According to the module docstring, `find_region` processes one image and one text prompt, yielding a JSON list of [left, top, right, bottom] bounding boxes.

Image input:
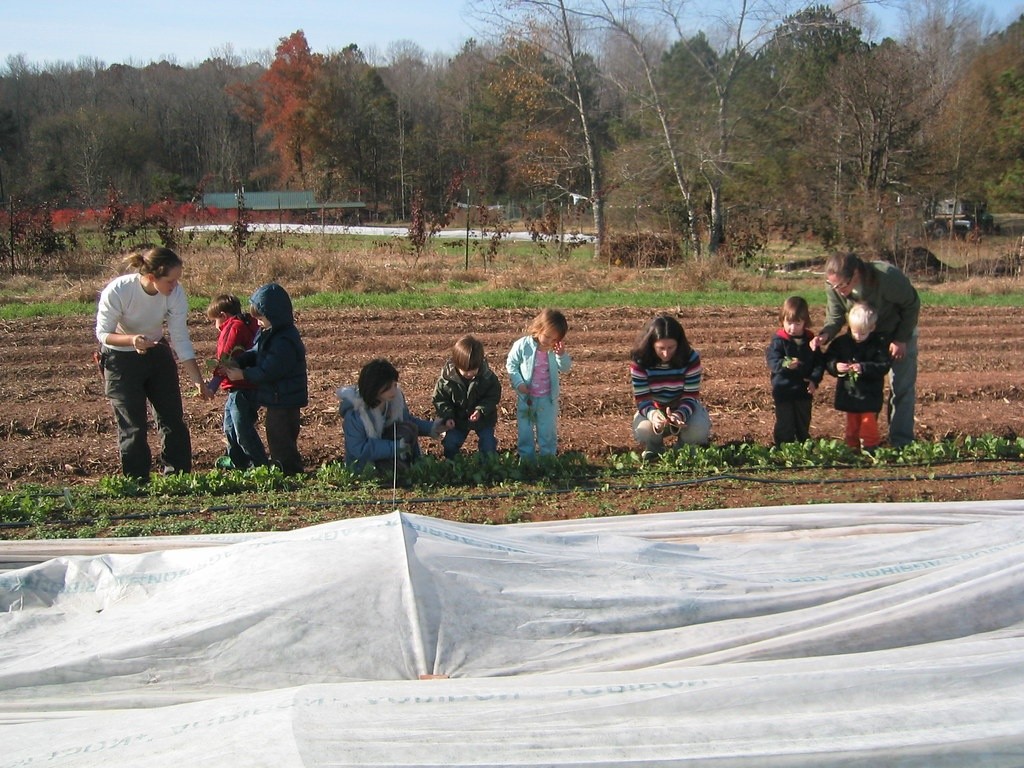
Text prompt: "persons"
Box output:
[[824, 303, 893, 448], [432, 337, 501, 460], [766, 296, 825, 452], [629, 316, 709, 458], [227, 284, 307, 478], [96, 248, 207, 480], [809, 252, 920, 449], [337, 358, 448, 472], [196, 295, 269, 469], [508, 310, 570, 460]]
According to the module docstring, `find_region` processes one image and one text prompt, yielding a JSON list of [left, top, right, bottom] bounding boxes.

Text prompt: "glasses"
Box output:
[[825, 274, 854, 289]]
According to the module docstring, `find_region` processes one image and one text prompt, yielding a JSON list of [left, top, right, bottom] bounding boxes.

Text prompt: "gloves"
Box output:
[[431, 418, 448, 433], [399, 437, 411, 455]]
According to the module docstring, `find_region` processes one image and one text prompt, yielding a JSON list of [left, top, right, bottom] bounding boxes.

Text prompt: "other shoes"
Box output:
[[214, 456, 238, 470], [862, 445, 881, 457], [848, 446, 860, 460]]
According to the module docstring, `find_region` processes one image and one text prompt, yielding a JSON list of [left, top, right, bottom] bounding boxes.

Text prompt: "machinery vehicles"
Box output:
[[917, 196, 1001, 238]]
